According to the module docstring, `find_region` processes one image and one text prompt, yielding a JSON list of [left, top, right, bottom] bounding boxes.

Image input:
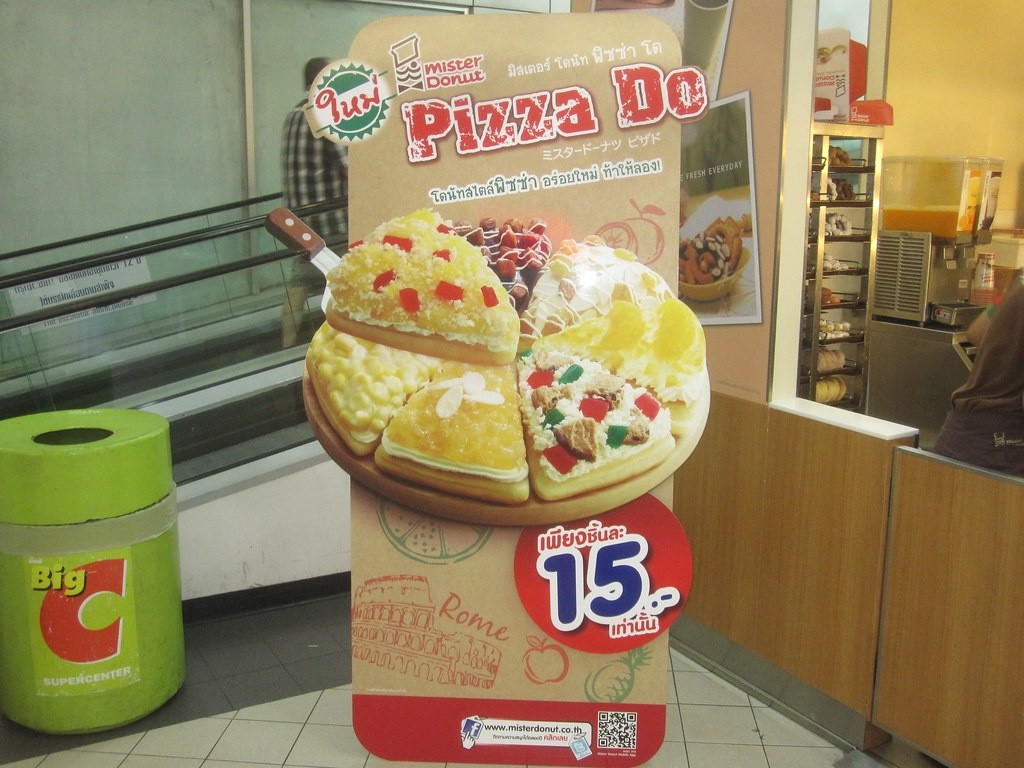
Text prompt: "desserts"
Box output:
[[679, 212, 752, 302]]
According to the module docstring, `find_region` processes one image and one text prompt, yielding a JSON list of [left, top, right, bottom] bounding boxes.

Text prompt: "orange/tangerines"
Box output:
[[959, 206, 976, 231]]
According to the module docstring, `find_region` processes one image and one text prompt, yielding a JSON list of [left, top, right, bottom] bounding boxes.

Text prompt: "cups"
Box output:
[[968, 252, 995, 309], [681, 0, 729, 72]]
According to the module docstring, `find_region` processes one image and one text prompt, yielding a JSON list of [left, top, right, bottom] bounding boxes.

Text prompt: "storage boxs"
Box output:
[[973, 234, 1024, 270]]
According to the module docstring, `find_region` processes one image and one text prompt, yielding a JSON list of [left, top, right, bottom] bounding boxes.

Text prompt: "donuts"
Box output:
[[816, 45, 848, 65], [799, 146, 854, 404]]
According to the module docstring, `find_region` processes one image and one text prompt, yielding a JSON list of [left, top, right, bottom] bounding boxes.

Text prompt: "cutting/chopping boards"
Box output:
[[301, 328, 712, 526]]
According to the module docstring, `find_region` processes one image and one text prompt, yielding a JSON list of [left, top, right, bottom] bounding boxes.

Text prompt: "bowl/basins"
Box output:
[[677, 247, 751, 301]]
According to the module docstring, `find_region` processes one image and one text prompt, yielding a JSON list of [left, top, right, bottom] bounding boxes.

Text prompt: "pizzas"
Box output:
[[306, 209, 708, 506]]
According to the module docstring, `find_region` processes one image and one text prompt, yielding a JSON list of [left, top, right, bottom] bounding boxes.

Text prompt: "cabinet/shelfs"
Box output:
[[796, 117, 886, 419]]
